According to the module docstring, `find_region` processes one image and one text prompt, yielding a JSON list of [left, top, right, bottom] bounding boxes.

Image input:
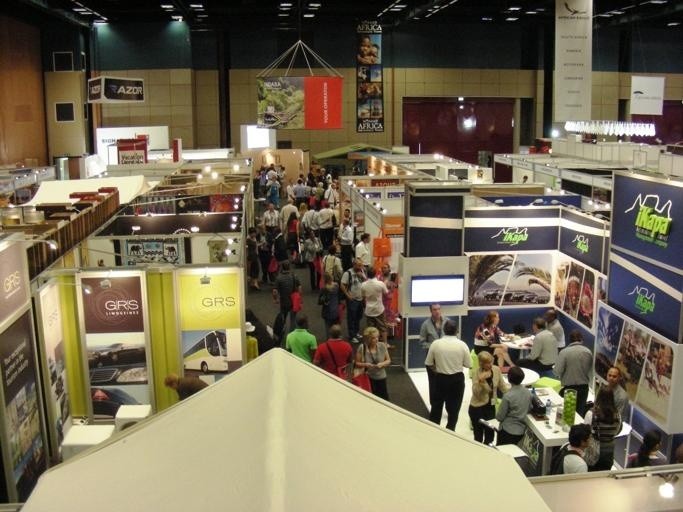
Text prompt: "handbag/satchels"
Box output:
[[337, 301, 348, 325], [267, 254, 280, 274], [336, 363, 351, 379], [350, 371, 372, 393], [331, 266, 343, 282], [290, 289, 303, 313]]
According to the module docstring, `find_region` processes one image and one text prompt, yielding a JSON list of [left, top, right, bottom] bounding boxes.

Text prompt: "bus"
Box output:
[[183, 329, 230, 377]]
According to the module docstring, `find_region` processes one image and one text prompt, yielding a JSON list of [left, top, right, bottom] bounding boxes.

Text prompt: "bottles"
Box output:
[[530, 387, 535, 394], [544, 399, 552, 416]]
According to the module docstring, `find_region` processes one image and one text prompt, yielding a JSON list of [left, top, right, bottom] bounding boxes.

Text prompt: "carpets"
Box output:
[[407, 368, 620, 470]]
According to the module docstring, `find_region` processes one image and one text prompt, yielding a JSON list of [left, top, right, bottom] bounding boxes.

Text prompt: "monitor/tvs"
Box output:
[[411, 274, 464, 306]]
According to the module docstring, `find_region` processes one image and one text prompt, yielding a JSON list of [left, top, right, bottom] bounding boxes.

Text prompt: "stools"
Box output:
[[615, 422, 632, 439], [491, 442, 529, 467], [479, 416, 500, 447]]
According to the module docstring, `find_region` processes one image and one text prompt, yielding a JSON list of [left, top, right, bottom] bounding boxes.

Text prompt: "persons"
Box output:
[[543, 308, 568, 349], [513, 317, 560, 374], [604, 365, 631, 422], [162, 374, 209, 401], [552, 328, 594, 418], [263, 99, 281, 124], [625, 429, 668, 469], [549, 422, 595, 478], [418, 300, 461, 414], [582, 388, 624, 473], [472, 310, 516, 368], [357, 33, 382, 123], [467, 350, 508, 445], [495, 367, 533, 448], [243, 157, 399, 405], [423, 319, 473, 433]]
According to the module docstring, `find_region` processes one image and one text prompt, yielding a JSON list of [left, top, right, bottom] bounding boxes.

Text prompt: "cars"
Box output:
[[483, 288, 539, 303], [88, 362, 149, 416], [49, 359, 68, 419]]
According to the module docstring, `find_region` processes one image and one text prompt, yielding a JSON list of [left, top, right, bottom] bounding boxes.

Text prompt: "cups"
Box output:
[[555, 407, 563, 425]]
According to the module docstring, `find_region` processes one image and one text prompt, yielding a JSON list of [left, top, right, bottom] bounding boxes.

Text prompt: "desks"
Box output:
[[518, 386, 584, 475], [504, 331, 537, 358]]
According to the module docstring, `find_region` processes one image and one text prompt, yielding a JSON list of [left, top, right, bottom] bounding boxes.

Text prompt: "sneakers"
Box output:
[[348, 332, 363, 345]]
